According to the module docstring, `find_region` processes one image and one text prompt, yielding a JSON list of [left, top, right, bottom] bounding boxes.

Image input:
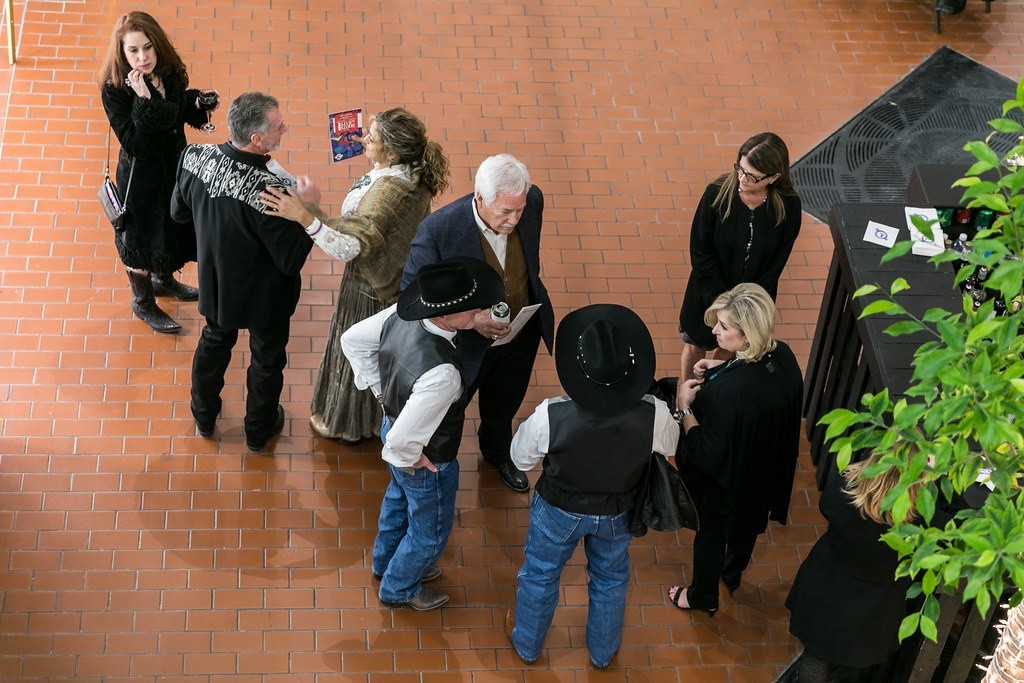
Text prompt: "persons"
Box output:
[[340, 255, 504, 612], [503, 304, 680, 670], [170, 93, 321, 453], [784, 422, 1000, 683], [402, 154, 555, 491], [668, 283, 804, 617], [100, 11, 220, 333], [257, 107, 449, 441], [678, 132, 801, 383]]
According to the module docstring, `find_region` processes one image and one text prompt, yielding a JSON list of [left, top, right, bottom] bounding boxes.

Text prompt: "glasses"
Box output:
[[734, 163, 771, 183]]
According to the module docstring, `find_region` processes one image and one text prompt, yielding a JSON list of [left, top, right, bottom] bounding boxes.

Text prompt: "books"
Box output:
[[328, 109, 365, 162]]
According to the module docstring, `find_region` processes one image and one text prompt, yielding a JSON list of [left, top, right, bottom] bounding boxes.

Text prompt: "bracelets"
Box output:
[[680, 408, 693, 419]]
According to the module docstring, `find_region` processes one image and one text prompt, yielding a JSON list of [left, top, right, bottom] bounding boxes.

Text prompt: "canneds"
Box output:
[[492, 301, 510, 318]]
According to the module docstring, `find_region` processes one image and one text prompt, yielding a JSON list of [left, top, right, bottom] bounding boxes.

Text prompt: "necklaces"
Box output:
[[155, 76, 160, 89], [738, 188, 767, 202]]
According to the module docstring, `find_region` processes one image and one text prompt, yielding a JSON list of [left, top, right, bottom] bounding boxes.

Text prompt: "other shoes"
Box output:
[[504, 608, 517, 640], [199, 431, 212, 437], [246, 405, 284, 451], [311, 414, 361, 446]]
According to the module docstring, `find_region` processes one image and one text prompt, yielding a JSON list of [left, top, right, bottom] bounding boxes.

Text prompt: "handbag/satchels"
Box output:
[[98, 178, 126, 229]]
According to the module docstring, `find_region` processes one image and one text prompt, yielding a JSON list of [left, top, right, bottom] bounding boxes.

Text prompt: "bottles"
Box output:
[[943, 232, 1020, 317]]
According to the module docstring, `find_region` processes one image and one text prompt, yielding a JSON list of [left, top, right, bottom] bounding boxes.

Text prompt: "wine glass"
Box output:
[[198, 88, 218, 134]]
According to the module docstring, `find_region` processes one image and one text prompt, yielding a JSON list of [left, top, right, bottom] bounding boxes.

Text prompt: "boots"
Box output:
[[150, 270, 199, 301], [126, 268, 182, 332]]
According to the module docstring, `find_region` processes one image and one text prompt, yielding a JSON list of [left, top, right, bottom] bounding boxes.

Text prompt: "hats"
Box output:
[[554, 304, 656, 416], [395, 256, 505, 322]]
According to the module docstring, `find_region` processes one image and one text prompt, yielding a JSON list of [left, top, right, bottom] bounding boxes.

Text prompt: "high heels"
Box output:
[[724, 582, 740, 596], [668, 585, 718, 617]]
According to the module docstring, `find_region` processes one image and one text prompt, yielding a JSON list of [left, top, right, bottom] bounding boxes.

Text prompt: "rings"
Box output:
[[492, 334, 497, 340]]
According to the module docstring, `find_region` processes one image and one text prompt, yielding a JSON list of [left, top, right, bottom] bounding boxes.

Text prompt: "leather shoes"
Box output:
[[372, 566, 441, 582], [500, 462, 531, 491], [406, 586, 449, 611]]
